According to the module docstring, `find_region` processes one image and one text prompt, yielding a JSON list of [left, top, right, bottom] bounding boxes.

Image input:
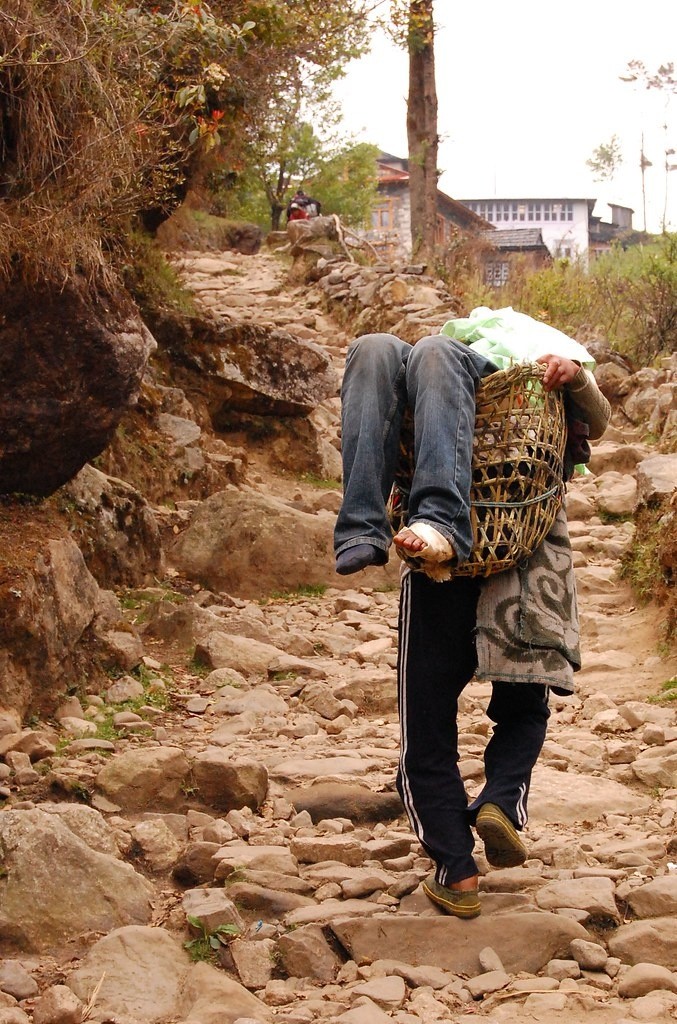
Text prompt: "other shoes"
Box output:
[[423, 871, 482, 919], [476, 802, 528, 868]]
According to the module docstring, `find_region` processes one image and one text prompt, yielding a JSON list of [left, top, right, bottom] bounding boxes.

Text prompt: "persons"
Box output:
[[287, 191, 322, 221], [334, 306, 612, 918]]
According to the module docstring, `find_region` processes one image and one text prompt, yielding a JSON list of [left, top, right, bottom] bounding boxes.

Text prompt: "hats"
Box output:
[[291, 202, 300, 209]]
